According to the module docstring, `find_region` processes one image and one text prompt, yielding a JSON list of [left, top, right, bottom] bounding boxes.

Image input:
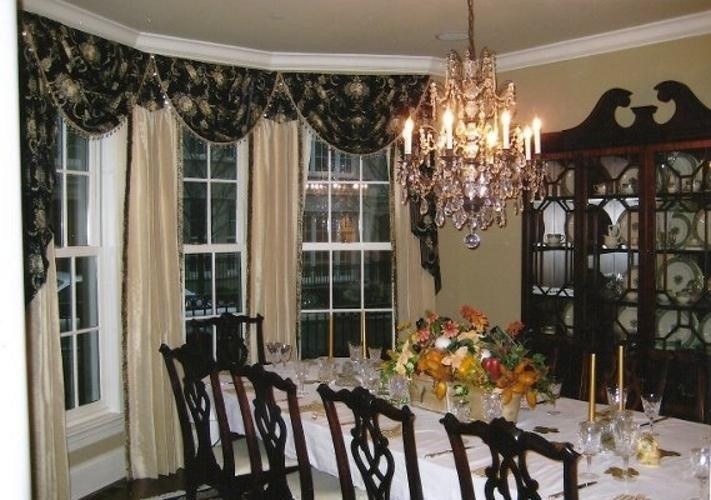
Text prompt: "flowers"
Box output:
[[374, 304, 562, 407]]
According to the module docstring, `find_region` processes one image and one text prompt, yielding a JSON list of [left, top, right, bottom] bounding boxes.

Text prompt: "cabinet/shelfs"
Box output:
[[521, 80, 711, 424]]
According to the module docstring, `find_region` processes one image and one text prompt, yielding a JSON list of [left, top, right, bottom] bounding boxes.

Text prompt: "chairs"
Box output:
[[159, 312, 710, 499]]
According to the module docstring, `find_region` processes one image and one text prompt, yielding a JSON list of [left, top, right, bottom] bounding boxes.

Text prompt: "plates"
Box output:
[[561, 152, 711, 350], [544, 242, 566, 247]]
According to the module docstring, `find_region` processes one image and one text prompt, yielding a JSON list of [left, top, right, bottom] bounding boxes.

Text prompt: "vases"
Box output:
[[409, 373, 523, 424]]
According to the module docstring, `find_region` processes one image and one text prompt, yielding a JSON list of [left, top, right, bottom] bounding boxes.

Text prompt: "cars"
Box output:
[[53, 269, 239, 351]]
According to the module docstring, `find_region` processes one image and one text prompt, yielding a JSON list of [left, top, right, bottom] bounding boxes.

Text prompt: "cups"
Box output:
[[451, 398, 471, 423], [481, 393, 503, 420], [367, 346, 382, 363], [548, 233, 567, 244]]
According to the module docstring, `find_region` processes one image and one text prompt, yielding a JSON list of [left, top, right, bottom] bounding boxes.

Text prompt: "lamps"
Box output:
[[395, 1, 547, 248]]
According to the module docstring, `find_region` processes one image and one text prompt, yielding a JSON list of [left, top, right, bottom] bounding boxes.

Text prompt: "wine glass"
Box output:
[[579, 423, 602, 480], [360, 365, 381, 397], [348, 343, 361, 375], [544, 381, 561, 415], [606, 385, 630, 422], [278, 344, 292, 374], [690, 448, 711, 500], [318, 357, 331, 384], [265, 342, 278, 371], [388, 377, 408, 408], [612, 421, 638, 481], [294, 360, 310, 397], [641, 393, 663, 436]]
